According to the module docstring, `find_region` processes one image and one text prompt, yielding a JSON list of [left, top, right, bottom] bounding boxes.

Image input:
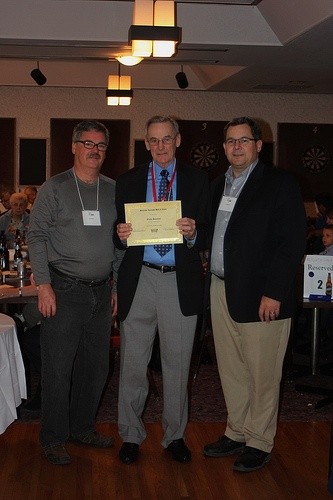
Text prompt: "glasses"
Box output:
[[225, 137, 255, 147], [146, 131, 179, 146], [73, 140, 106, 151]]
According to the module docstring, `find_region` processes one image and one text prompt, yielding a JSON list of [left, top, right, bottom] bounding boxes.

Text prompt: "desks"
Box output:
[[0, 267, 41, 303], [0, 309, 30, 441]]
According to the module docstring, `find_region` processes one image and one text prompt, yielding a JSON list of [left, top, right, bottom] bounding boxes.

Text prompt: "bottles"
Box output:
[[0, 230, 9, 270], [14, 229, 23, 271]]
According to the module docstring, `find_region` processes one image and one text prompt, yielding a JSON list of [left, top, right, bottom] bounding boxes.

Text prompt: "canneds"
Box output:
[[17, 261, 25, 276]]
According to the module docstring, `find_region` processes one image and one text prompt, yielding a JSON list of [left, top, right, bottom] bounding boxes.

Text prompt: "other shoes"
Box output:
[[169, 438, 192, 462], [202, 435, 246, 457], [233, 446, 272, 472], [39, 439, 72, 465], [70, 427, 115, 448], [119, 442, 139, 464]]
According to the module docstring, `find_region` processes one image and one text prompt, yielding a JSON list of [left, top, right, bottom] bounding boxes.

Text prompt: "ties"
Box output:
[[153, 169, 173, 257]]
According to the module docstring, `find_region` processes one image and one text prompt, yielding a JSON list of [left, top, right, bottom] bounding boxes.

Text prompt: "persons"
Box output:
[[306, 192, 333, 254], [209, 117, 306, 472], [27, 120, 117, 465], [112, 115, 214, 463], [0, 184, 37, 249]]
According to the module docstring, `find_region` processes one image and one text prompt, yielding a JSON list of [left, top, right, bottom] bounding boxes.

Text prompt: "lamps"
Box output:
[[114, 55, 144, 66], [128, 0, 182, 57], [105, 57, 132, 107], [30, 59, 47, 85], [175, 64, 188, 89]]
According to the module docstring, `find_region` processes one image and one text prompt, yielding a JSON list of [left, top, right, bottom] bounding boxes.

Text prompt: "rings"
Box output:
[[270, 312, 275, 314]]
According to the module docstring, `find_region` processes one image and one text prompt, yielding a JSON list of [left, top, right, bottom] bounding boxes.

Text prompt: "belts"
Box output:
[[211, 273, 226, 280], [142, 261, 175, 274], [47, 264, 110, 285]]
